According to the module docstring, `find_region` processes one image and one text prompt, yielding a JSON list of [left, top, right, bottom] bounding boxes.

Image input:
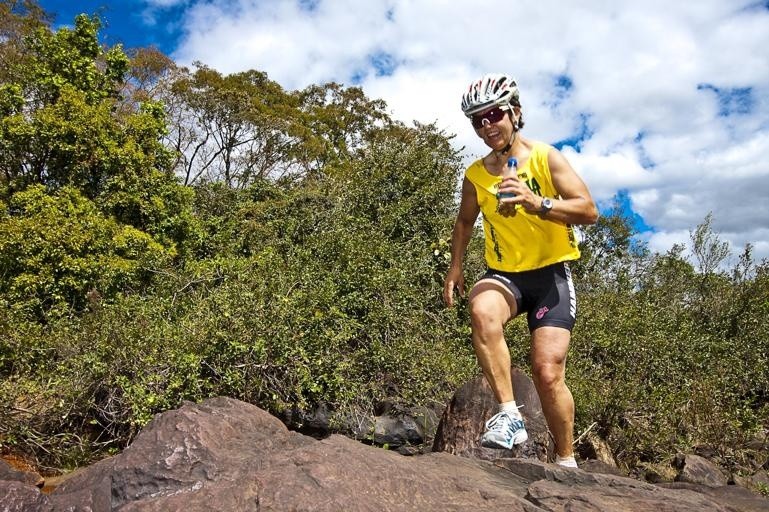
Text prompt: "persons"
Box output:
[[444, 74, 600, 469]]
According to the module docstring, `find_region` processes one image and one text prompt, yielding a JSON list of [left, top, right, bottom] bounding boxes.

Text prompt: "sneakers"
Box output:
[[480, 412, 529, 451]]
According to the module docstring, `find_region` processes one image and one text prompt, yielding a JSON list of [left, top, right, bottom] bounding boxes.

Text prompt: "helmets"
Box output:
[[460, 72, 520, 116]]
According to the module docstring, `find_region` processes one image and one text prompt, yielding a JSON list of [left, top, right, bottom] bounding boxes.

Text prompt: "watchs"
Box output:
[[539, 195, 554, 219]]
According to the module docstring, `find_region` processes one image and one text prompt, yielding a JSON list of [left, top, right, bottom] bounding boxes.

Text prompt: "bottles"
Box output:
[[496, 157, 518, 217]]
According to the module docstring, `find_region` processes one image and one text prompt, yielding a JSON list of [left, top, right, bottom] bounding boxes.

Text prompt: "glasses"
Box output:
[[471, 106, 515, 129]]
